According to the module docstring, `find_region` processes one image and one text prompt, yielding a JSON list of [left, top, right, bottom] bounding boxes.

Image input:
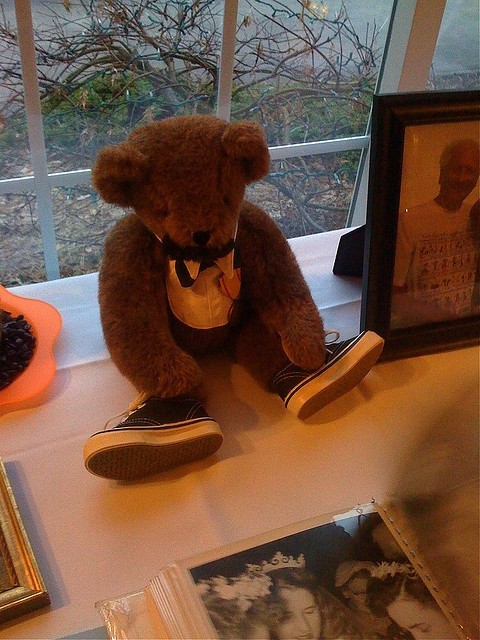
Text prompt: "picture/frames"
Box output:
[[0, 462, 52, 621], [332, 88, 480, 359]]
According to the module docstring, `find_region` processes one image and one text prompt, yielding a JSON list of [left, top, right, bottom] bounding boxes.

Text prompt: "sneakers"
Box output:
[[268, 329, 385, 421], [82, 390, 223, 481]]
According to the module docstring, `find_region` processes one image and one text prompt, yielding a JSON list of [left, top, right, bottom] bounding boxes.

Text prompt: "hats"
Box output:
[[334, 562, 377, 588]]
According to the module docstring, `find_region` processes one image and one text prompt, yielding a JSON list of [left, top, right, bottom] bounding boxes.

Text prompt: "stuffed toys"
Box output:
[[81, 113, 387, 483]]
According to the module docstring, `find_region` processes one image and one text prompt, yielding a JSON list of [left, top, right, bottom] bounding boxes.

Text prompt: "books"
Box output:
[[93, 499, 475, 640]]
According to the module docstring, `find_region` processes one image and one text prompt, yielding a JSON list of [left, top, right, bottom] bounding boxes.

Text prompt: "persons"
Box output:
[[244, 549, 377, 640], [388, 139, 480, 328], [367, 561, 460, 639], [332, 558, 412, 640], [195, 572, 277, 640]]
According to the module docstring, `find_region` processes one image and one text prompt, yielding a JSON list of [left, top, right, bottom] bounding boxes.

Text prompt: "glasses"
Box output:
[[347, 587, 367, 597]]
